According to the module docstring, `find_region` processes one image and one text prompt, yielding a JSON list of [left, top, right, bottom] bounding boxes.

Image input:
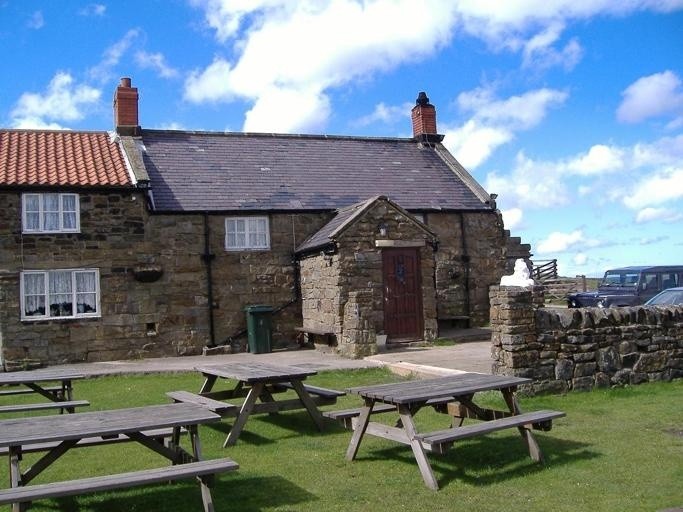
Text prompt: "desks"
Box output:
[[0, 368, 84, 413], [193, 359, 330, 449], [0, 402, 221, 488], [346, 373, 541, 489]]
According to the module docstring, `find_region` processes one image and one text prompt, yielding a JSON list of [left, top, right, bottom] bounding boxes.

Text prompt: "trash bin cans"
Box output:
[[244, 305, 273, 353]]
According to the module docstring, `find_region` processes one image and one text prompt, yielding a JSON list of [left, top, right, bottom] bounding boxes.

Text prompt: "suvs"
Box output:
[[566, 264, 683, 309]]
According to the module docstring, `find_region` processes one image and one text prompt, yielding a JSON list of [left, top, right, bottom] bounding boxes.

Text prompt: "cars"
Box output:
[[644, 286, 683, 306]]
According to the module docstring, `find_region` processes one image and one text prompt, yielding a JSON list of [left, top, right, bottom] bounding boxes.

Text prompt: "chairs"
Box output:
[[647, 278, 657, 288], [664, 279, 675, 289], [631, 276, 639, 282]]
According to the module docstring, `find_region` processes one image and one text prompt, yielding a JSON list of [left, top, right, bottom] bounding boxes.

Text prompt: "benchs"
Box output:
[[1, 387, 67, 395], [322, 396, 457, 430], [413, 408, 568, 456], [166, 390, 237, 420], [0, 400, 90, 414], [273, 383, 346, 403], [1, 426, 188, 460], [1, 456, 239, 512]]
